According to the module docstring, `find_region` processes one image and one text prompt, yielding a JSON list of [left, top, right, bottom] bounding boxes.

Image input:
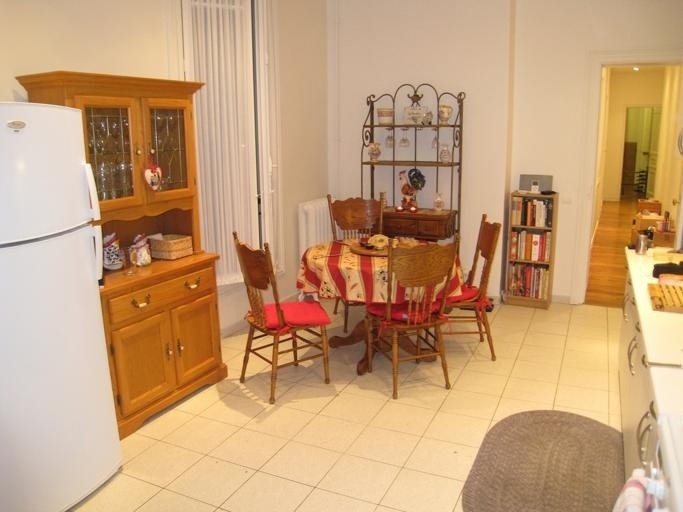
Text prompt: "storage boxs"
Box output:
[[636, 199, 661, 215], [636, 212, 664, 231], [652, 226, 675, 248], [631, 228, 649, 248]]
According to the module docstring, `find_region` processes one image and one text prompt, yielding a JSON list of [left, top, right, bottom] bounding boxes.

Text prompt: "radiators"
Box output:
[[299, 198, 366, 301]]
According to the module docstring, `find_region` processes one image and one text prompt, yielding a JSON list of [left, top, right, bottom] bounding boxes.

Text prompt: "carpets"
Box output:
[[462, 410, 624, 512]]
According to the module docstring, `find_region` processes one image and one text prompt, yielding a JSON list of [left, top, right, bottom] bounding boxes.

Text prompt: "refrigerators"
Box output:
[[0, 101, 126, 512]]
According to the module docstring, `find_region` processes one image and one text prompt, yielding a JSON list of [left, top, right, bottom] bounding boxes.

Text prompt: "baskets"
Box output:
[[150, 234, 193, 261]]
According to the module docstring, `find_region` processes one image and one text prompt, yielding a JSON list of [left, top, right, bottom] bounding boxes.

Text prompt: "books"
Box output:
[[507, 197, 553, 300]]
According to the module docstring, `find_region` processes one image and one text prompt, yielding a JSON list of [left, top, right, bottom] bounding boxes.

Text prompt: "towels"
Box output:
[[611, 475, 656, 512], [611, 469, 667, 512]]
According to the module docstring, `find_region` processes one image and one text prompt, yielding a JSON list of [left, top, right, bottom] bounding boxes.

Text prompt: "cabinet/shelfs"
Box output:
[[619, 246, 683, 512], [358, 83, 464, 243], [100, 253, 226, 441], [502, 192, 559, 310], [15, 71, 205, 270]]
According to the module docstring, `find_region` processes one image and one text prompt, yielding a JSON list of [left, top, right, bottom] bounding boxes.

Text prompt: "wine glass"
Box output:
[[386, 128, 392, 147], [399, 128, 408, 147], [432, 128, 439, 148]]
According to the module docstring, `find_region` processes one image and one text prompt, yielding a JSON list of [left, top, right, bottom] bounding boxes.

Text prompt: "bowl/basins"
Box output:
[[360, 242, 367, 246], [366, 244, 373, 249]]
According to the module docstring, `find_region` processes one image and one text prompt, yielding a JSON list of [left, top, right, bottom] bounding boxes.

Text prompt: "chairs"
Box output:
[[233, 233, 330, 403], [327, 193, 398, 333], [367, 236, 457, 399], [439, 213, 502, 361]]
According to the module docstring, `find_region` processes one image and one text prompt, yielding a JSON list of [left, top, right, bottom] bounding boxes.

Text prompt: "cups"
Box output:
[[392, 239, 399, 248], [636, 236, 647, 254], [121, 246, 136, 276]]
